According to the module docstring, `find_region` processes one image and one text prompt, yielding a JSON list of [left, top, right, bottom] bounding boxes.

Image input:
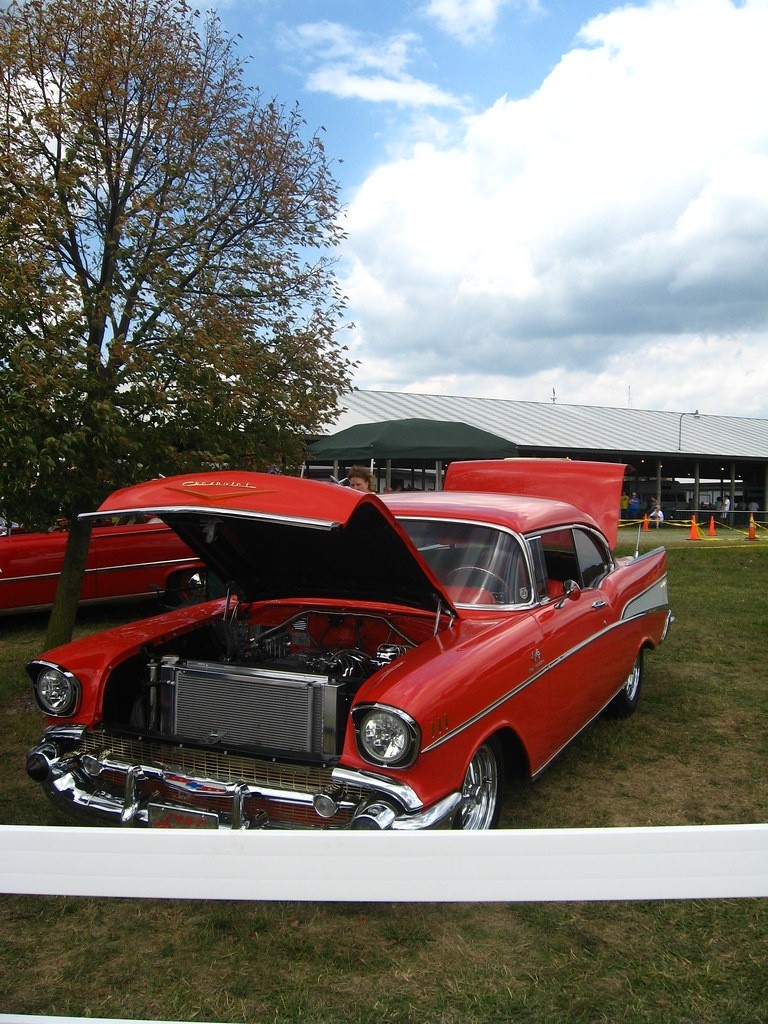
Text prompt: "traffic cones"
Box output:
[[706, 513, 719, 537], [685, 514, 703, 541], [745, 513, 761, 542], [640, 512, 652, 532]]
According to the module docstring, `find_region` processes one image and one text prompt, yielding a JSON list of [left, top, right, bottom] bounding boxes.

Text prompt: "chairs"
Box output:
[[480, 550, 527, 603]]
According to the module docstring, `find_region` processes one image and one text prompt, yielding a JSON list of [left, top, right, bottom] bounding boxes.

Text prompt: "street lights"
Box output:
[[678, 409, 700, 452]]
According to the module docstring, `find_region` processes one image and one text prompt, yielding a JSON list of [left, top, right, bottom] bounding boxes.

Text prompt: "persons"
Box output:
[[382, 485, 760, 526], [349, 466, 373, 494]]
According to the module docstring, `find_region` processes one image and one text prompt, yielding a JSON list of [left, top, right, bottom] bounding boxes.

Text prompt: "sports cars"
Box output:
[[0, 457, 210, 613], [26, 455, 675, 831]]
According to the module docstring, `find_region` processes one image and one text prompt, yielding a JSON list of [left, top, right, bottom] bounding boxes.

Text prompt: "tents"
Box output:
[[305, 419, 519, 493]]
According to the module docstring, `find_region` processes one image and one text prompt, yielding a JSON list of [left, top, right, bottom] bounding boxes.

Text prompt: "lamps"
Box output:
[[679, 410, 700, 450]]
